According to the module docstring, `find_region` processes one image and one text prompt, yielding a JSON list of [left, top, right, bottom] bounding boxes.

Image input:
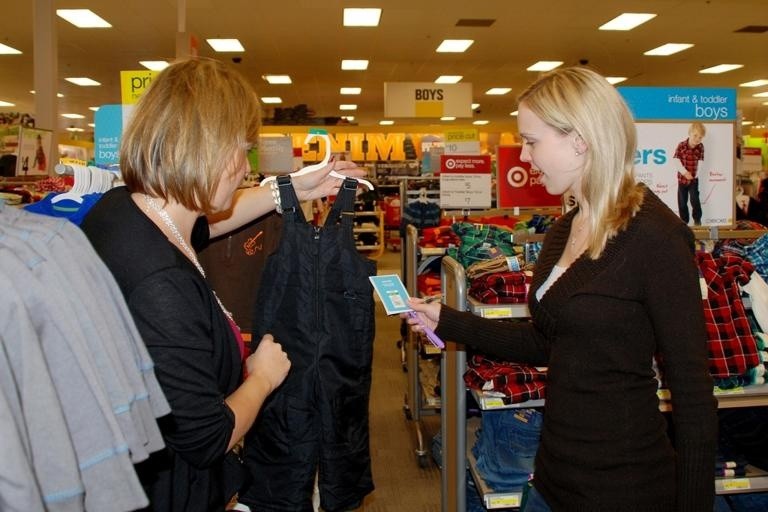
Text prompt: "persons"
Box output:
[[397, 61, 721, 512], [672, 122, 707, 225], [31, 133, 46, 171], [76, 54, 367, 512]]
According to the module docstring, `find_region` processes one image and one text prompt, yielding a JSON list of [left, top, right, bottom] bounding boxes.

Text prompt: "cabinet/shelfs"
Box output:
[[399, 214, 768, 509], [307, 164, 442, 262], [734, 171, 767, 203]]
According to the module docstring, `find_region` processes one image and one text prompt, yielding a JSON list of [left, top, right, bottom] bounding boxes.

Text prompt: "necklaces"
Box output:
[[137, 192, 233, 319], [568, 217, 590, 252]]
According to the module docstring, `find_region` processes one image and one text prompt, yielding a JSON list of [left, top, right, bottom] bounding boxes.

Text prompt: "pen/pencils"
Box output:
[[408, 312, 445, 350]]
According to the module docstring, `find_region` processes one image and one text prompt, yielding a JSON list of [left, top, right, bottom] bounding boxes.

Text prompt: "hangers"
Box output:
[[259, 128, 379, 192], [45, 163, 115, 209]]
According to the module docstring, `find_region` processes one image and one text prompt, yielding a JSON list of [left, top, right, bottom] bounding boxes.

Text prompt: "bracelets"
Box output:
[[268, 178, 283, 215]]
